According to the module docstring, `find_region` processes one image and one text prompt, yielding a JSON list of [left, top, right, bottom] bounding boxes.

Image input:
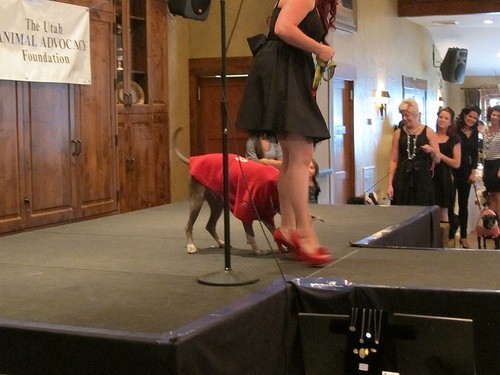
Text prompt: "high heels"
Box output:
[[459, 238, 471, 249], [274, 227, 306, 261], [291, 232, 332, 266]]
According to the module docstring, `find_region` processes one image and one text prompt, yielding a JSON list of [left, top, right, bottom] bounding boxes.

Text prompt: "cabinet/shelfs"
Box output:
[[0, 0, 170, 234]]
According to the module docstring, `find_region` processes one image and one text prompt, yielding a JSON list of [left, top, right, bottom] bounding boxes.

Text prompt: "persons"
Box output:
[[455, 105, 479, 249], [307, 158, 320, 203], [481, 105, 500, 218], [435, 107, 461, 222], [385, 98, 440, 206], [235, 0, 337, 266], [242, 133, 283, 169]]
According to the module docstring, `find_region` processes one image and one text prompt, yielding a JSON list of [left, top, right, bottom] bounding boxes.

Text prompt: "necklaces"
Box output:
[[404, 127, 421, 160]]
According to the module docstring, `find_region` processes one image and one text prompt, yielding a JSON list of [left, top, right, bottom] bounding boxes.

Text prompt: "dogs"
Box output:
[[172, 125, 325, 256], [476, 209, 500, 250], [474, 189, 491, 209], [347, 190, 392, 206]]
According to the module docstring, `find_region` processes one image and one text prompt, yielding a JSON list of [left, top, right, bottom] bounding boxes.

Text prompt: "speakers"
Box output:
[[440, 48, 468, 84], [168, 0, 211, 21]]
